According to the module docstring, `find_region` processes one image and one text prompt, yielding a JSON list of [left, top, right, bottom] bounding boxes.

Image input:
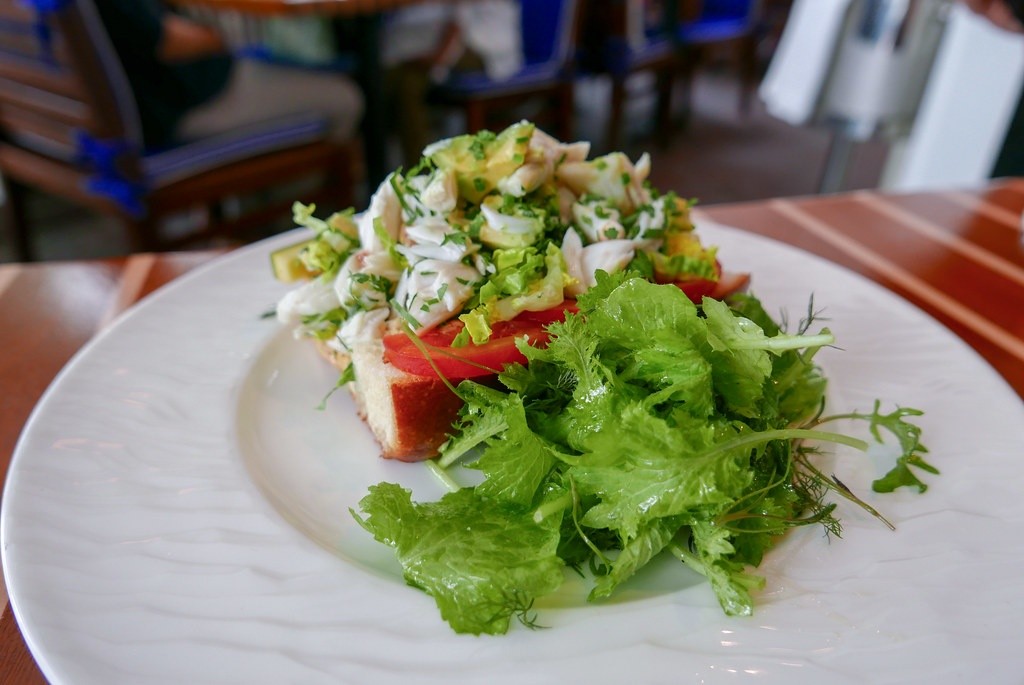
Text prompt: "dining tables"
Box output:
[[0, 179, 1024, 684]]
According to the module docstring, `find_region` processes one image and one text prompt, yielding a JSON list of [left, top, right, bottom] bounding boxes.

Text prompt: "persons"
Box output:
[[386, 0, 579, 175], [90, 0, 361, 235]]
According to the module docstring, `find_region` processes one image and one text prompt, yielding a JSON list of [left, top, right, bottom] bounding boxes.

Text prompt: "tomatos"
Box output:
[[379, 320, 551, 378], [514, 302, 587, 325], [653, 273, 753, 304]]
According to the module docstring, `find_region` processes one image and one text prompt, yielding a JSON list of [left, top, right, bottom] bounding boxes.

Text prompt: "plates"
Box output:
[[0, 213, 1024, 685]]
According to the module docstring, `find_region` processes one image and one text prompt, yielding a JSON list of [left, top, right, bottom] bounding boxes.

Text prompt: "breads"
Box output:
[[342, 339, 515, 462]]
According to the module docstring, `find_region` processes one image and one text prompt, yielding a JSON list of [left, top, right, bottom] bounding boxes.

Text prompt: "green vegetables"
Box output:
[[288, 126, 716, 405], [351, 268, 941, 640]]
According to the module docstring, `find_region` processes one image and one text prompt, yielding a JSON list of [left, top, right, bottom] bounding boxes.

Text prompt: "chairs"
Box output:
[[0, 0, 363, 251], [368, 0, 764, 172]]
